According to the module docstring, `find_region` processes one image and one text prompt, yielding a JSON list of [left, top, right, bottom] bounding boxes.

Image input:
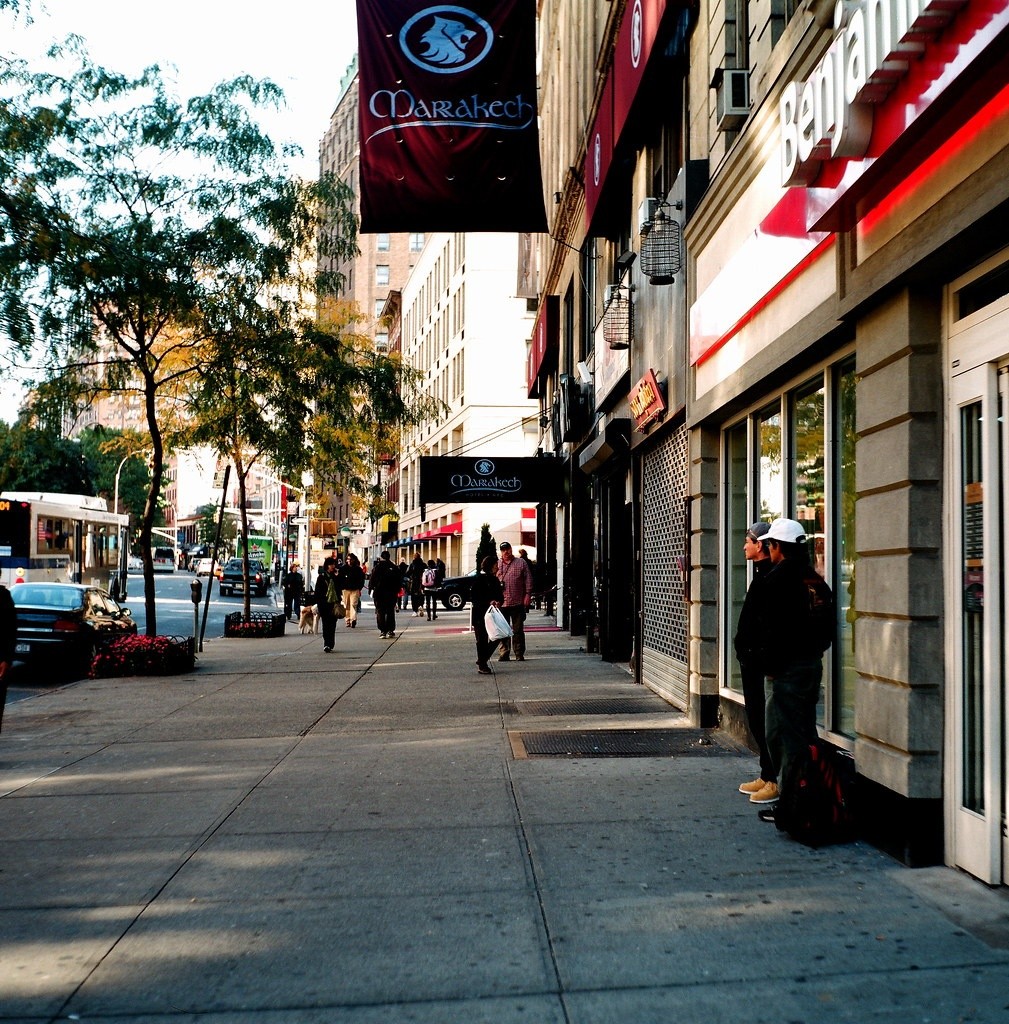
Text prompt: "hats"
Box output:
[[757, 517, 806, 543], [500, 542, 511, 550], [745, 521, 771, 541], [382, 551, 390, 560]]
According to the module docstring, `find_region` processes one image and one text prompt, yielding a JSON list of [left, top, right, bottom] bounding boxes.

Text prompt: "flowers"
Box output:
[[87, 633, 189, 680], [229, 620, 272, 639]]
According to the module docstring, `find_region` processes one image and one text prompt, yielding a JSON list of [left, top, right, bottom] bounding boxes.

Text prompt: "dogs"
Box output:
[[298, 604, 321, 636]]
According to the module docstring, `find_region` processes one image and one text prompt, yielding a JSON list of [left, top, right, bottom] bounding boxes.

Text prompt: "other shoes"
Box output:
[[758, 809, 775, 822], [427, 617, 431, 621], [476, 659, 479, 665], [418, 606, 424, 617], [432, 615, 438, 620], [324, 646, 330, 653], [379, 632, 386, 639], [412, 612, 418, 617], [351, 620, 356, 628], [386, 631, 395, 638], [346, 623, 350, 627], [498, 656, 510, 661], [478, 667, 491, 673], [516, 656, 524, 661]]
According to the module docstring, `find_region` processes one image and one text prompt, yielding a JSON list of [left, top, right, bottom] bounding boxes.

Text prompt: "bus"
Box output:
[[0, 492, 130, 603]]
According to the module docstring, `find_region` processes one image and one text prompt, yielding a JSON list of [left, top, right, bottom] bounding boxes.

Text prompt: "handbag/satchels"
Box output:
[[333, 603, 346, 619], [485, 605, 514, 642]]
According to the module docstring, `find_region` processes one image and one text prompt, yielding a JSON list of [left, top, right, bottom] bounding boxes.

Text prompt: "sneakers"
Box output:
[[739, 778, 765, 795], [749, 781, 780, 804]]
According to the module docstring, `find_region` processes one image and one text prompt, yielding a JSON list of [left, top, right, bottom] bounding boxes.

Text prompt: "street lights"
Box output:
[[289, 535, 296, 564], [341, 527, 351, 563]]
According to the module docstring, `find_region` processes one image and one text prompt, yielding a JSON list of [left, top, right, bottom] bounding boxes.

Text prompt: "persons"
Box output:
[[471, 557, 507, 674], [495, 543, 531, 661], [370, 549, 447, 639], [315, 555, 343, 653], [518, 549, 532, 573], [283, 563, 304, 620], [701, 518, 835, 804], [340, 552, 363, 628], [0, 567, 18, 730]]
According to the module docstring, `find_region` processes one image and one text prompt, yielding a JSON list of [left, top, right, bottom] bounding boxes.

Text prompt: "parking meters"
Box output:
[[190, 580, 203, 653]]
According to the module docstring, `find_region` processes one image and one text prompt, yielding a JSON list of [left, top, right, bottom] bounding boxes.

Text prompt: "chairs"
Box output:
[[26, 591, 45, 604]]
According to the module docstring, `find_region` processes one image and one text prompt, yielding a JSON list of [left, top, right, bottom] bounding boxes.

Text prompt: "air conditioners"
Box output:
[[637, 197, 658, 236], [716, 70, 751, 133]]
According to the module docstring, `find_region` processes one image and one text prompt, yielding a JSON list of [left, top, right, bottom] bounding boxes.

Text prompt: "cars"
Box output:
[[436, 568, 486, 611], [188, 558, 269, 597], [7, 582, 137, 681]]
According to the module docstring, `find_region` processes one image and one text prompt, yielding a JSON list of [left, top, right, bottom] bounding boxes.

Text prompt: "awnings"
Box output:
[[386, 521, 462, 548]]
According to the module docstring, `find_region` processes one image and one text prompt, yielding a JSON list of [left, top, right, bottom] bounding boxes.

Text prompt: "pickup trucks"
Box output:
[[152, 547, 174, 572]]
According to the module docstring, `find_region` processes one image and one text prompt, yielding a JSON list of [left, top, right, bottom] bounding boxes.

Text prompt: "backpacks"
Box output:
[[421, 568, 435, 587], [775, 744, 856, 848]]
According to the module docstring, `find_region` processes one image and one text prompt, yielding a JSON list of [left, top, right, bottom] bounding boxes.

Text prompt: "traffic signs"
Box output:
[[288, 515, 308, 525]]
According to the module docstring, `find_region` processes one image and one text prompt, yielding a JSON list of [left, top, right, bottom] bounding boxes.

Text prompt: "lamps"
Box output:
[[638, 200, 683, 286], [602, 284, 635, 351]]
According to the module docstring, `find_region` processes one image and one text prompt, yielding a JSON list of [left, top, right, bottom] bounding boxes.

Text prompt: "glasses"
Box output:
[[745, 529, 758, 540]]
